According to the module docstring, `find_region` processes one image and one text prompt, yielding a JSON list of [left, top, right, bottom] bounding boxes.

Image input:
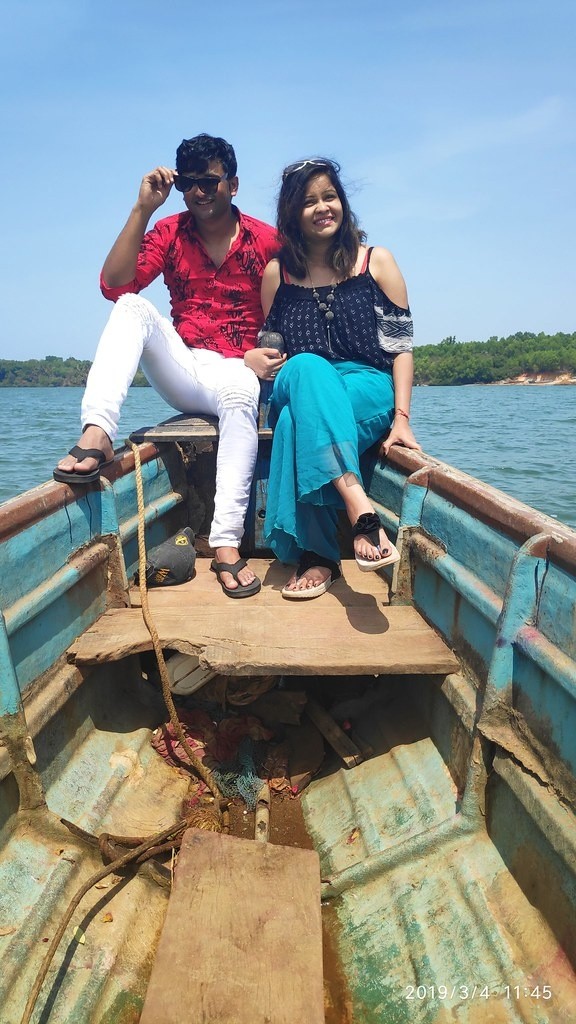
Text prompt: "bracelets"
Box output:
[[394, 407, 410, 420]]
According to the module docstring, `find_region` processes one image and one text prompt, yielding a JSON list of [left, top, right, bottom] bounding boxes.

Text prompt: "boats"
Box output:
[[0, 412, 574, 1022]]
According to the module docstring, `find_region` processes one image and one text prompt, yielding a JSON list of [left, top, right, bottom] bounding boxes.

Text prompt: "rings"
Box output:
[[270, 372, 276, 378]]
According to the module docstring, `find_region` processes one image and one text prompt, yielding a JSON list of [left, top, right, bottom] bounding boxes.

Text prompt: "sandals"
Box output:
[[282, 554, 342, 598], [52, 445, 114, 483], [351, 512, 401, 572], [211, 556, 261, 598]]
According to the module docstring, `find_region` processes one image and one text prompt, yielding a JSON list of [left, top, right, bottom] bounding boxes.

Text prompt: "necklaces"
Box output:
[[303, 259, 340, 352]]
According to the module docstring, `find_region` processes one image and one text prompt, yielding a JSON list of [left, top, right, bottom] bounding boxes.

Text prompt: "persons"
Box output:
[[243, 157, 424, 598], [53, 132, 285, 598]]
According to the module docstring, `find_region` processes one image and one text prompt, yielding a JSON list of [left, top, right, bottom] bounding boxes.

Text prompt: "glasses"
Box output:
[[282, 160, 333, 181], [173, 169, 229, 194]]
